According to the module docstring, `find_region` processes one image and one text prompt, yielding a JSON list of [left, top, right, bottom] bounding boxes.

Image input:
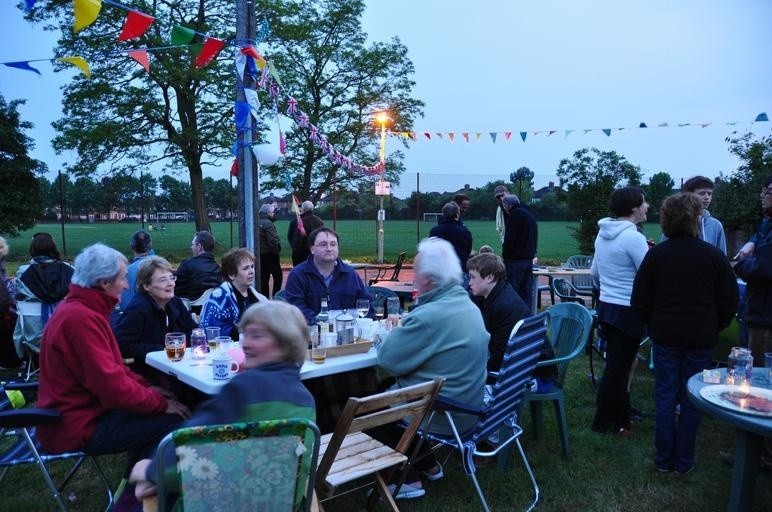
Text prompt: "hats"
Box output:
[[495, 186, 507, 197]]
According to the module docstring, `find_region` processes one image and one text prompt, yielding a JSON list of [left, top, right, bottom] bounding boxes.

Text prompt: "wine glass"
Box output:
[[373, 306, 384, 329], [356, 298, 370, 318]]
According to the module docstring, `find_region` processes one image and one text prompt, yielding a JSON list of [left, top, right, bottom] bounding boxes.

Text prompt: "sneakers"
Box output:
[[593, 408, 644, 436], [474, 454, 498, 466]]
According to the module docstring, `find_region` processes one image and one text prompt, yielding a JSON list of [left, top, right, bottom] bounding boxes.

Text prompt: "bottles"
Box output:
[[316, 296, 334, 333], [726, 346, 753, 397], [190, 328, 207, 360]]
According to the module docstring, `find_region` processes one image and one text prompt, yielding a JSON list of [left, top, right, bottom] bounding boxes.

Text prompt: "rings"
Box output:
[[175, 400, 179, 407]]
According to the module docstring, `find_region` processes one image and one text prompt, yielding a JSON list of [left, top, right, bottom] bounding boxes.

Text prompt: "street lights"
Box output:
[[373, 110, 389, 261]]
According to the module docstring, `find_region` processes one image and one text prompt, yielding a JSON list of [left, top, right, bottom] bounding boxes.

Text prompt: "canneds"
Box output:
[[728, 346, 753, 379], [387, 296, 400, 315]]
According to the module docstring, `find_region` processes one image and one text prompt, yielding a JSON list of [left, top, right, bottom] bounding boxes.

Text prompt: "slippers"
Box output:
[[424, 461, 443, 480], [367, 483, 425, 501]]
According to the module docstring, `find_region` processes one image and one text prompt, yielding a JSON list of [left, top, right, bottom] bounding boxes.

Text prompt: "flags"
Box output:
[[258, 70, 384, 176]]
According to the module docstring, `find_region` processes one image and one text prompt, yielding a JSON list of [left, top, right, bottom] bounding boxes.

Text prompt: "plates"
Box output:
[[699, 384, 771, 416]]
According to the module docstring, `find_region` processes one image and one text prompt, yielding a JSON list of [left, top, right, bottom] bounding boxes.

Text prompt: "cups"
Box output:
[[765, 352, 771, 380], [165, 332, 187, 364], [214, 336, 234, 353], [212, 356, 240, 379], [387, 296, 399, 326], [311, 331, 327, 363], [206, 326, 222, 352], [325, 334, 337, 346]]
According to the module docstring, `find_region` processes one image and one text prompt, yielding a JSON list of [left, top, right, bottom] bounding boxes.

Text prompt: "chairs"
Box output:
[[0, 252, 600, 512]]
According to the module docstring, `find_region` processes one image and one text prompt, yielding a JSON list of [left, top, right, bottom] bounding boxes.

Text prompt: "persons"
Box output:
[[258, 203, 282, 299], [36, 243, 194, 490], [427, 184, 540, 307], [466, 253, 558, 469], [590, 187, 651, 438], [286, 201, 324, 267], [658, 176, 726, 256], [114, 255, 210, 407], [632, 191, 740, 474], [728, 177, 771, 366], [283, 228, 375, 392], [0, 212, 274, 372], [129, 300, 310, 512], [367, 237, 491, 499]]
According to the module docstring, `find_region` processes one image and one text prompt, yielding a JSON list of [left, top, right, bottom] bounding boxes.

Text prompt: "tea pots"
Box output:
[[335, 309, 362, 345]]
